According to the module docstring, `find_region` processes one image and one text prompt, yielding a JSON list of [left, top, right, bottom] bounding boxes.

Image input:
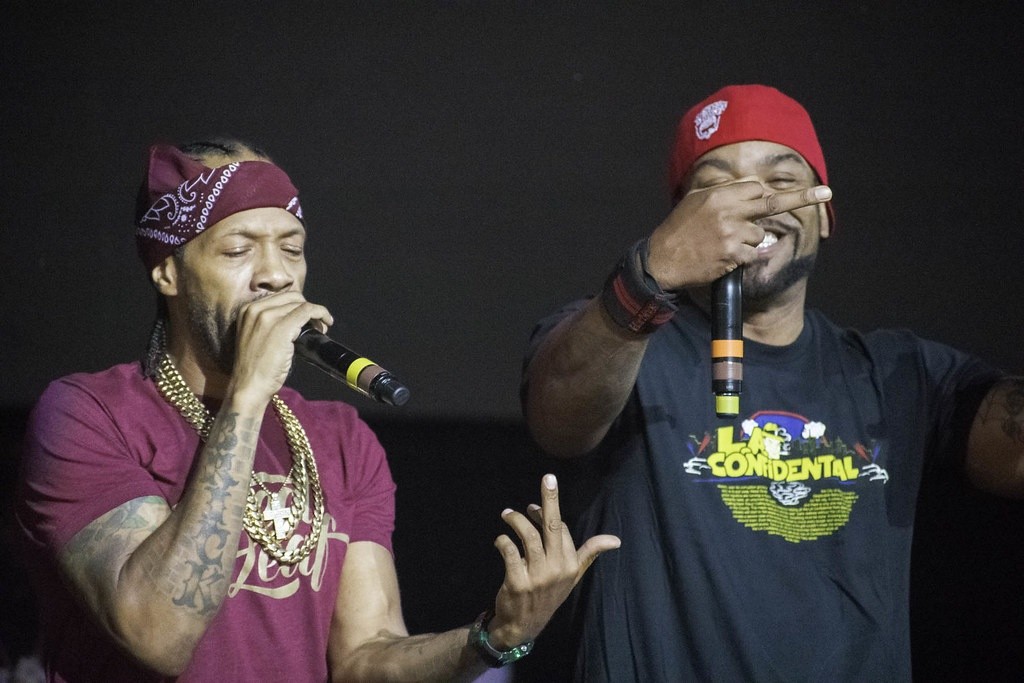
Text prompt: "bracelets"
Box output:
[[602, 237, 689, 335]]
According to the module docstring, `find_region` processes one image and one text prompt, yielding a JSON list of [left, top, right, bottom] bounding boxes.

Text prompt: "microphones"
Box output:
[[710, 263, 748, 418], [293, 324, 411, 406]]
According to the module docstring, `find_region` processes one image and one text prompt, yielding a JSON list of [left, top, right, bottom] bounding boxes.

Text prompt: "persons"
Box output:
[[14, 139, 622, 683], [520, 85, 1024, 683]]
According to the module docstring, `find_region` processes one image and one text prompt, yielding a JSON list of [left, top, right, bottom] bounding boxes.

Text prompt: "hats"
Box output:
[[667, 85, 838, 238]]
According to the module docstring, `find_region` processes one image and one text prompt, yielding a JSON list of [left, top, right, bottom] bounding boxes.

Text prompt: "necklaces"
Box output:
[[154, 352, 324, 566]]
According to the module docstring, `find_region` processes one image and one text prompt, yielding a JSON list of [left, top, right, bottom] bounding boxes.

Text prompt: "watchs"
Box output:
[[472, 610, 535, 668]]
